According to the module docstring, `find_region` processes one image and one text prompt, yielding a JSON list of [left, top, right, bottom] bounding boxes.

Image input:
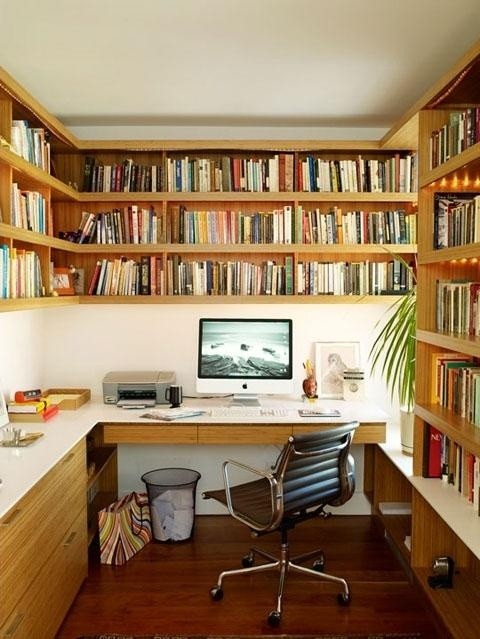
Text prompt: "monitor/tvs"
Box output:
[[194, 317, 294, 399]]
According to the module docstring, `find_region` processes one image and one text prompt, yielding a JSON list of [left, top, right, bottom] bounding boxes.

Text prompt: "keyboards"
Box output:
[[209, 406, 291, 419]]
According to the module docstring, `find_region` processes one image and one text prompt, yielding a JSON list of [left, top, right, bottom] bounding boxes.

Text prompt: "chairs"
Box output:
[[200, 422, 360, 628]]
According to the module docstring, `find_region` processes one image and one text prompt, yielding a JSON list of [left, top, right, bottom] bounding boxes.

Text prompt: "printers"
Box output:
[[103, 372, 176, 408]]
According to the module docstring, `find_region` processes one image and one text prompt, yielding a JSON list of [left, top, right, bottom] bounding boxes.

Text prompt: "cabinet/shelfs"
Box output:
[[0, 434, 90, 638]]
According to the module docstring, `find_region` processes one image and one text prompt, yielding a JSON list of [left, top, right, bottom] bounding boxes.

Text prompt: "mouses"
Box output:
[[315, 407, 333, 414]]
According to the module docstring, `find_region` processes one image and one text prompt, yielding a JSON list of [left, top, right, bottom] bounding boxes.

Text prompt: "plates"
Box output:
[[2, 433, 44, 446]]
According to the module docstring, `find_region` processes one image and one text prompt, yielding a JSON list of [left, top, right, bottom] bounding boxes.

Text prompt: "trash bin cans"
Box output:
[[141, 468, 201, 544]]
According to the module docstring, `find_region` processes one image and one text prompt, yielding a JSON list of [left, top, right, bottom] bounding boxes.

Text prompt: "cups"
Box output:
[[343, 368, 364, 402], [165, 385, 183, 408], [0, 428, 21, 443]]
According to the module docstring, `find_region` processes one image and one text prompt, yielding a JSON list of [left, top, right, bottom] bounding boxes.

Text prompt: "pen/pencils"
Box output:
[[303, 359, 314, 378]]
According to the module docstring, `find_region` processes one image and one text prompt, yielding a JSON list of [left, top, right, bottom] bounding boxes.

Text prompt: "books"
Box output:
[[8, 404, 59, 424], [140, 405, 206, 422], [296, 152, 416, 296], [7, 396, 52, 414], [168, 153, 295, 294], [0, 119, 49, 298], [75, 156, 164, 295], [417, 91, 478, 510], [298, 405, 342, 419]]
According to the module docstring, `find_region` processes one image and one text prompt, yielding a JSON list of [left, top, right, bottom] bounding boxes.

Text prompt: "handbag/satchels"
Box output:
[[96, 491, 154, 567]]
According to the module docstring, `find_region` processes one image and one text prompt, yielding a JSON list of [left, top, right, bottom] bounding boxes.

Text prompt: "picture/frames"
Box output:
[[314, 341, 361, 400]]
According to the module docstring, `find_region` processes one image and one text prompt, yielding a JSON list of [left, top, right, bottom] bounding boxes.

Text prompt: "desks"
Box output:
[[1, 395, 386, 547]]
